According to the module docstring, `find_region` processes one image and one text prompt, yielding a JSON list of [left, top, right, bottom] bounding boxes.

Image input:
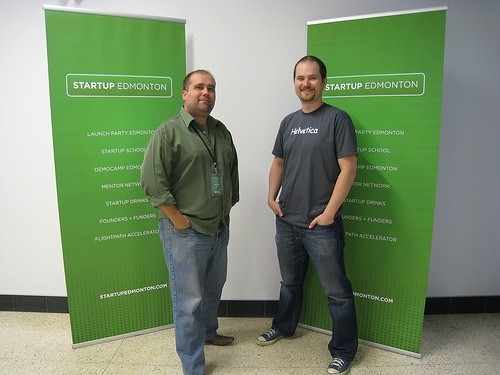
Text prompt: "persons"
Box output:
[[258, 56, 359, 374], [142, 70, 239, 374]]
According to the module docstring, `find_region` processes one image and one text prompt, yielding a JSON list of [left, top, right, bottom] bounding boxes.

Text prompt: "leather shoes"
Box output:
[[202, 334, 234, 346]]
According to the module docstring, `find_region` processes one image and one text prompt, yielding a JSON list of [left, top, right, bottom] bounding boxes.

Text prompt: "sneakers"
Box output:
[[327, 356, 352, 375], [255, 328, 284, 346]]
